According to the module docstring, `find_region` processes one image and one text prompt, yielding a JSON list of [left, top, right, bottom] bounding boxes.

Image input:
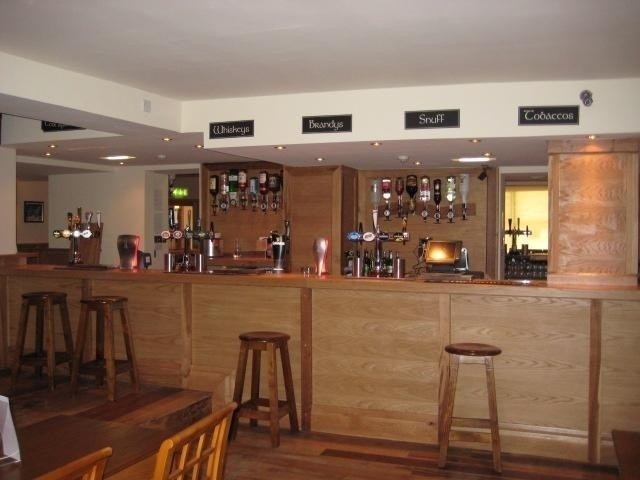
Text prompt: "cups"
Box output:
[[163, 252, 176, 271], [392, 258, 405, 278], [205, 238, 215, 259], [273, 241, 286, 268], [195, 253, 207, 272], [303, 264, 317, 280]]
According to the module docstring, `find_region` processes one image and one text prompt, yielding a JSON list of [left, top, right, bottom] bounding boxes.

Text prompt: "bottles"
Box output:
[[209, 168, 281, 216], [368, 174, 470, 223], [345, 248, 399, 276], [232, 240, 241, 258]]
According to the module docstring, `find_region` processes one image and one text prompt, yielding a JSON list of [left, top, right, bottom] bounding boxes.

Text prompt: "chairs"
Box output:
[[34, 447, 113, 479], [104, 402, 238, 480]]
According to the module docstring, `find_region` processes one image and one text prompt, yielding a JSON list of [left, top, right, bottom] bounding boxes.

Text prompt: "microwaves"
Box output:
[[425, 239, 463, 264]]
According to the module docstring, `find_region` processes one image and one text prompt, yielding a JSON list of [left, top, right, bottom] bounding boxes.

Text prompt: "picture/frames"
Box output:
[[24, 201, 44, 224]]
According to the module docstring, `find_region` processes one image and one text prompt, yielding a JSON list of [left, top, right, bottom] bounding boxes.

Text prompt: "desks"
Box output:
[[1, 415, 179, 480], [612, 430, 640, 480]]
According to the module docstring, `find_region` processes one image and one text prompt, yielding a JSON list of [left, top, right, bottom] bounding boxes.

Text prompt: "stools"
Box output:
[[439, 343, 502, 472], [230, 331, 299, 447], [12, 291, 78, 392], [70, 296, 140, 402]]
